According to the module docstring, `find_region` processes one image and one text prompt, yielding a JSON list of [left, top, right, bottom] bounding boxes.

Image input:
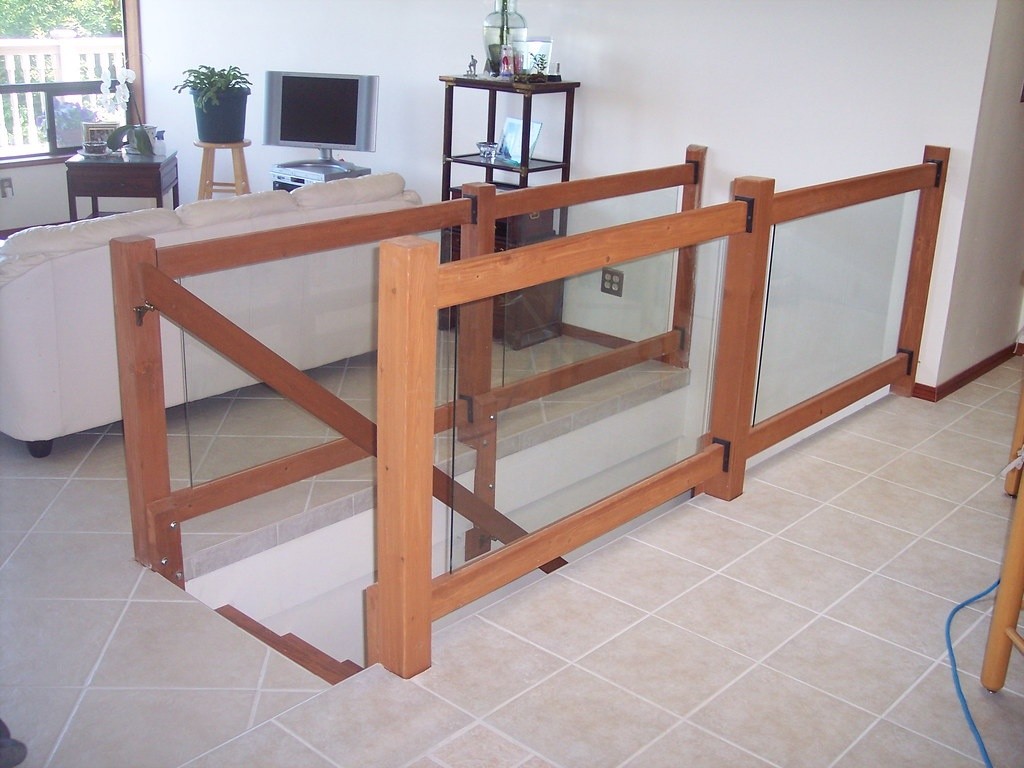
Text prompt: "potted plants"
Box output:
[[172, 65, 253, 143], [37, 97, 104, 147]]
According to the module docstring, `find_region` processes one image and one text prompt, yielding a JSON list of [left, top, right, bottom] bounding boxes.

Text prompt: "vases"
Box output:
[[128, 124, 156, 149]]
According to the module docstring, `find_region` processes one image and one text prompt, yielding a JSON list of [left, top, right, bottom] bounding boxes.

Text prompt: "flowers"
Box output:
[[99, 53, 157, 156]]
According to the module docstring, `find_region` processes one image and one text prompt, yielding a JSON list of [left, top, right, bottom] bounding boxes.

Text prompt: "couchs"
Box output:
[[0, 172, 421, 458]]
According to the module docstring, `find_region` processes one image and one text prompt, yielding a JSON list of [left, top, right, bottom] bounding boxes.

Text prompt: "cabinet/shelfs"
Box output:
[[439, 74, 582, 351]]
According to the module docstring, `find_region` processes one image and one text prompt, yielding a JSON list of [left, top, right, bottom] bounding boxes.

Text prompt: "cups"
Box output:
[[83, 141, 107, 154], [477, 142, 498, 158]]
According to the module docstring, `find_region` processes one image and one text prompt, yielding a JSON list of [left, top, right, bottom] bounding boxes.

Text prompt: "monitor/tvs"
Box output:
[[261, 71, 379, 172]]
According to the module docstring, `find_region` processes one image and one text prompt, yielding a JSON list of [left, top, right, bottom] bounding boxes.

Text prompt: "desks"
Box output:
[[194, 138, 252, 200], [65, 150, 180, 220]]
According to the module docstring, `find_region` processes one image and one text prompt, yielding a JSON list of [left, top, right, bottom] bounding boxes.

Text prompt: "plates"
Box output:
[[77, 149, 112, 157]]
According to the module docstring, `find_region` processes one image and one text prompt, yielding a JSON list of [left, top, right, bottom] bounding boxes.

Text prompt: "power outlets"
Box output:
[[601, 267, 624, 297]]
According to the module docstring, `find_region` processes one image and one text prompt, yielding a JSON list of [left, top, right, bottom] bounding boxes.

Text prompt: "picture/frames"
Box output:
[[495, 116, 544, 168], [82, 122, 120, 142]]
